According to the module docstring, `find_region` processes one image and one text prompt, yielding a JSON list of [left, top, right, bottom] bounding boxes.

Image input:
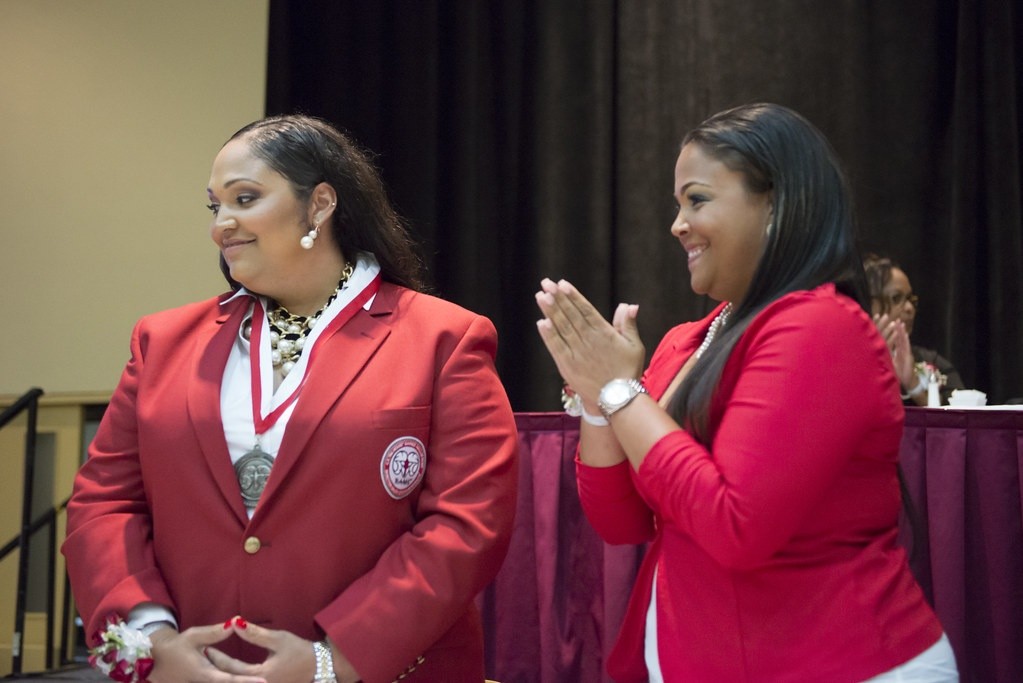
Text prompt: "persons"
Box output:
[[59, 117, 521, 683], [856, 253, 966, 407], [535, 102, 960, 683]]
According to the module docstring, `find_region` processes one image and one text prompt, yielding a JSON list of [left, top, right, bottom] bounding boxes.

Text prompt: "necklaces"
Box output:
[[695, 302, 732, 360], [245, 263, 354, 378]]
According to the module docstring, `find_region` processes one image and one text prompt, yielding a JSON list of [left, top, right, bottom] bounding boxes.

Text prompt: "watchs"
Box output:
[[597, 378, 647, 420]]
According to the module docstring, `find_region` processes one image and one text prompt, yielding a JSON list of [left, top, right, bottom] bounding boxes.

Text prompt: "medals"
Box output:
[[232, 450, 275, 508]]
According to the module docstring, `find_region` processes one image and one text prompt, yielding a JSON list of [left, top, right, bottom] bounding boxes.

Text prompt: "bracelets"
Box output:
[[908, 362, 946, 395], [91, 613, 168, 683], [562, 385, 609, 426], [312, 641, 337, 682], [900, 394, 911, 400]]
